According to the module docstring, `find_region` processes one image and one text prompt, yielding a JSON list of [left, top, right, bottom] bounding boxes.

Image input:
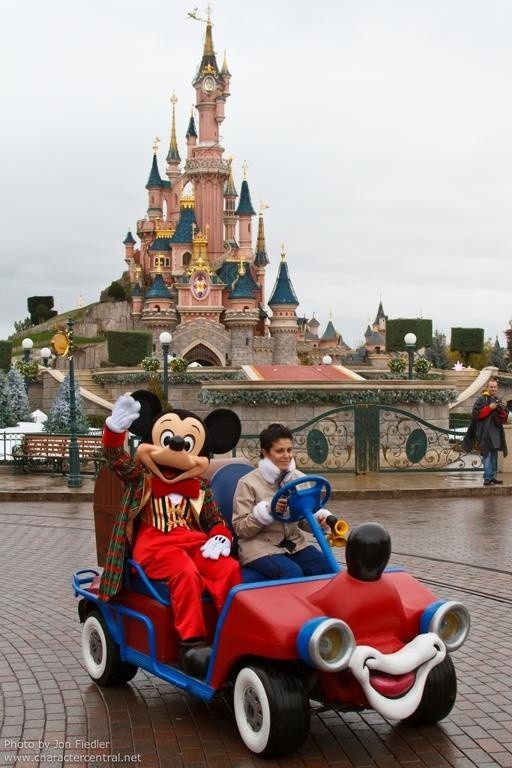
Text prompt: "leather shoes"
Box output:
[[484, 478, 502, 486]]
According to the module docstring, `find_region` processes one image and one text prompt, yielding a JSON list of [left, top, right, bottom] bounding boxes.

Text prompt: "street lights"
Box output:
[[22, 337, 34, 361], [40, 347, 51, 368], [159, 329, 173, 406], [403, 332, 418, 380]]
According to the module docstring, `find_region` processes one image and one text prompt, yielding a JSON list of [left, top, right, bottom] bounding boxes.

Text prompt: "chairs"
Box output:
[[121, 457, 322, 599]]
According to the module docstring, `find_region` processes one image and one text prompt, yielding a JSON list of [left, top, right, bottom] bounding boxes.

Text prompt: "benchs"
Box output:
[[11, 435, 107, 482]]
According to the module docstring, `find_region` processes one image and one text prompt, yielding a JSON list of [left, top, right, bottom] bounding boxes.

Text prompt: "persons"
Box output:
[[472, 379, 509, 485], [102, 390, 243, 676], [231, 423, 331, 580]]
